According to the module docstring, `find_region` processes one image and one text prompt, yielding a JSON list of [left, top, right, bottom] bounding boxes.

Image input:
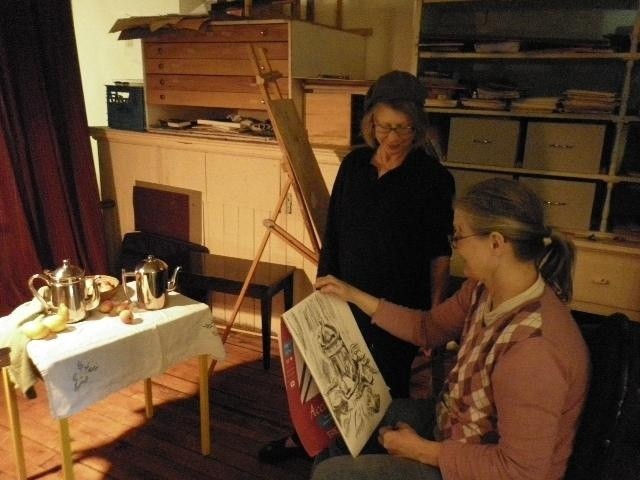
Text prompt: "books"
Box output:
[[420, 28, 621, 118]]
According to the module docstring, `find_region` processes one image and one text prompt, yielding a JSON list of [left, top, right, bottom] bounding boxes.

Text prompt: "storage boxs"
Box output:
[[448, 117, 606, 232]]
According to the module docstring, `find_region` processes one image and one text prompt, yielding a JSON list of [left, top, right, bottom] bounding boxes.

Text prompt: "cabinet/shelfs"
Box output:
[[140, 19, 369, 139], [90, 125, 351, 343], [408, 2, 640, 243], [448, 232, 640, 448]]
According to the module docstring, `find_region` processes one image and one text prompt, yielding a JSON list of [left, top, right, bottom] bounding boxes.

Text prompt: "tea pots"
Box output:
[[121, 254, 183, 311], [27, 259, 102, 324]]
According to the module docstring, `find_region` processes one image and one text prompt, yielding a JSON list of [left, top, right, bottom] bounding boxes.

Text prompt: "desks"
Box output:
[[0, 280, 211, 480]]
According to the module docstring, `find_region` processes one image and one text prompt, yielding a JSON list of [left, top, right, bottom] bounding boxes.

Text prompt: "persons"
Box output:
[[259, 68, 457, 462], [312, 176, 593, 480]]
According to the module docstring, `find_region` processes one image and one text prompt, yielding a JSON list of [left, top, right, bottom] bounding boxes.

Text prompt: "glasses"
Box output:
[[373, 123, 417, 136]]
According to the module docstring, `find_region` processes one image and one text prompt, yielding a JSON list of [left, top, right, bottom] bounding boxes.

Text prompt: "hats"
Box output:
[[364, 71, 426, 116]]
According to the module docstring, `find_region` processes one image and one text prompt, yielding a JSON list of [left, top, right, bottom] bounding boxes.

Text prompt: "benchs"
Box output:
[[121, 243, 296, 370]]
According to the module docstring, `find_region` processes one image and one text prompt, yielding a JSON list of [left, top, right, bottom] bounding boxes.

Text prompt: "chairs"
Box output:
[[563, 313, 636, 480]]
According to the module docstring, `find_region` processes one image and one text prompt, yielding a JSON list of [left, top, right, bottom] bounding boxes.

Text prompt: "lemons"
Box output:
[[21, 303, 70, 339], [98, 300, 133, 323]]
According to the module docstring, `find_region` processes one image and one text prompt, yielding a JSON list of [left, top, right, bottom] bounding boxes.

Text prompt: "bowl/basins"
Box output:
[[86, 275, 120, 300]]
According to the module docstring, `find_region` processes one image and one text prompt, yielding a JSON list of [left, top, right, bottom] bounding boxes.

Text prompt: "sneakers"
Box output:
[[258, 435, 304, 462]]
